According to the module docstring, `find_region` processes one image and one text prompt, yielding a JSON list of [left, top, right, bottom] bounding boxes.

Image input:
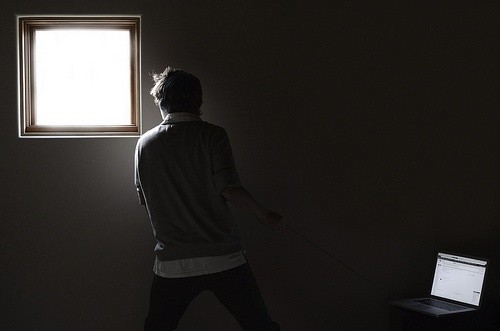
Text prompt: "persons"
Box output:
[[137, 71, 281, 331]]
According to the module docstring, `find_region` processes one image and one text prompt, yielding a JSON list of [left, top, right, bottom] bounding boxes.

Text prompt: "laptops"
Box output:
[[389, 250, 490, 318]]
